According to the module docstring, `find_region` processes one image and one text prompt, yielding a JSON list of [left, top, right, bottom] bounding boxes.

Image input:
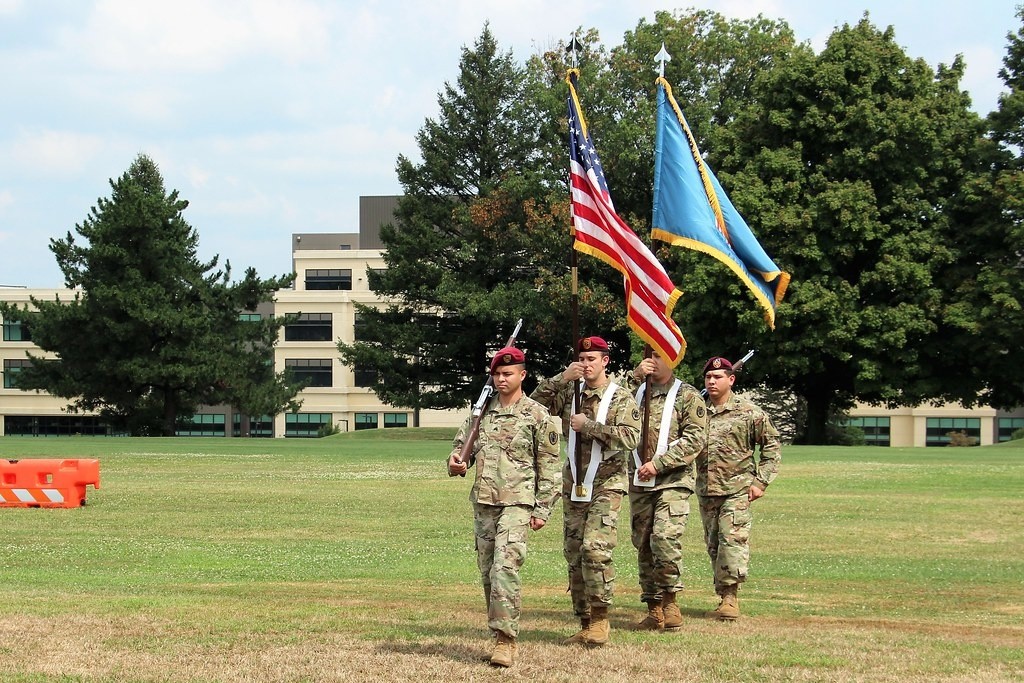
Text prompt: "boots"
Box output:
[[637, 601, 664, 630], [490, 629, 511, 667], [662, 592, 683, 628], [481, 631, 519, 661], [587, 605, 611, 643], [563, 617, 590, 644], [719, 585, 739, 620], [705, 589, 723, 619]]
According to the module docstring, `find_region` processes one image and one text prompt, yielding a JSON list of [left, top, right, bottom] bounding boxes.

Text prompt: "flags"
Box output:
[[650, 76, 791, 330], [564, 67, 687, 370]]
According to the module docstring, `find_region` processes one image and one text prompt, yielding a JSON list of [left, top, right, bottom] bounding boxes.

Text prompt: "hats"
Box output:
[[702, 356, 732, 375], [490, 346, 525, 375], [576, 337, 608, 355]]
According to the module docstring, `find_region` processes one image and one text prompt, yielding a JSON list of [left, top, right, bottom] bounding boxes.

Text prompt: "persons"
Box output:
[[529, 336, 642, 645], [695, 355, 782, 619], [615, 346, 707, 631], [446, 346, 560, 667]]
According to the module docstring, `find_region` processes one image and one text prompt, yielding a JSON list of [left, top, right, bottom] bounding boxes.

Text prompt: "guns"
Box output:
[[458, 317, 526, 470], [700, 350, 758, 397]]
